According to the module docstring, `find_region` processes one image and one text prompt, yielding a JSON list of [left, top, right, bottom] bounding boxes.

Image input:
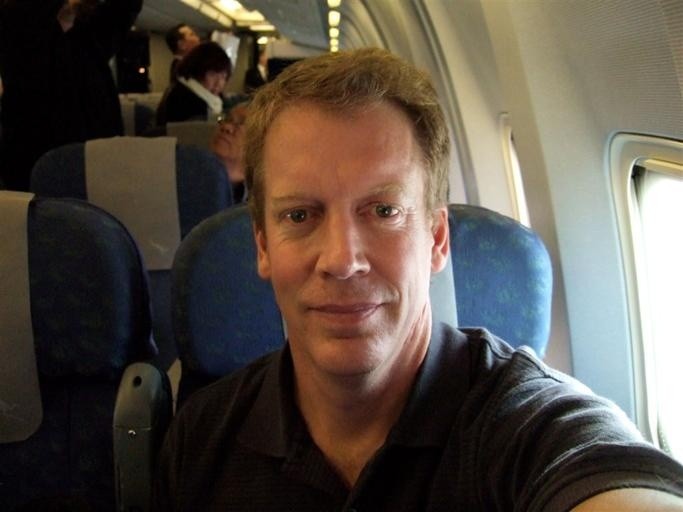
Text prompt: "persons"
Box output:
[[0, 1, 144, 191], [144, 46, 683, 511], [242, 47, 275, 92], [164, 21, 202, 88], [153, 40, 234, 130]]
